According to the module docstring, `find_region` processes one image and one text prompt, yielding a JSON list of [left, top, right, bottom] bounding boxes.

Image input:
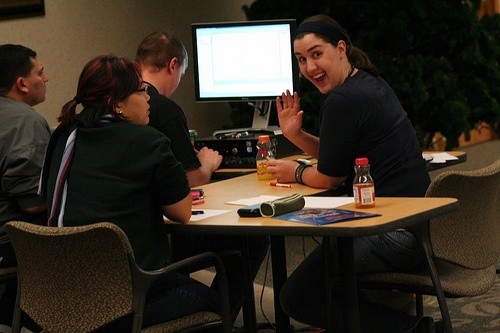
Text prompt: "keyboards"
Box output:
[[218, 156, 256, 169]]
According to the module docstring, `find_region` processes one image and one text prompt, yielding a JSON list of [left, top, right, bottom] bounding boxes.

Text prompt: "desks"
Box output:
[[163, 155, 461, 333]]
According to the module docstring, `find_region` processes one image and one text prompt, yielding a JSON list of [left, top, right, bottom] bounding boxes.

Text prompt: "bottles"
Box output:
[[187, 130, 198, 147], [353, 158, 375, 208], [256, 135, 276, 180]]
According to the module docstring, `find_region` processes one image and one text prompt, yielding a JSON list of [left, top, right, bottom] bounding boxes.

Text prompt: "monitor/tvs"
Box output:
[[191, 19, 301, 135]]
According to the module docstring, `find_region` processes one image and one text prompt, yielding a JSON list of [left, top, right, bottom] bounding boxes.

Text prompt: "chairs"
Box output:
[[358, 158, 500, 333], [2, 219, 232, 333]]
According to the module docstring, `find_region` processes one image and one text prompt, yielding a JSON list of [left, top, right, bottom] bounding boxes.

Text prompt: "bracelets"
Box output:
[[295, 164, 313, 184]]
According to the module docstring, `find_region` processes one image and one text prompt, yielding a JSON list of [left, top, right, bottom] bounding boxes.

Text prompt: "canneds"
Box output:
[[188, 130, 198, 147]]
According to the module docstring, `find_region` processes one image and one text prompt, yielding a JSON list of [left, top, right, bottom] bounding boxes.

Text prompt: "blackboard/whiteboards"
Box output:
[[0, 0, 45, 21]]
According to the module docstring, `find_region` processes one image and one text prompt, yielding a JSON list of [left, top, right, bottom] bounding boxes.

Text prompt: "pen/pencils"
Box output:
[[192, 196, 204, 200], [194, 148, 199, 154], [256, 145, 270, 161], [191, 210, 204, 215], [270, 182, 292, 188]]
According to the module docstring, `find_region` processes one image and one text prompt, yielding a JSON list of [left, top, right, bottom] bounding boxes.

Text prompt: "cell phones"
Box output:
[[237, 204, 261, 217]]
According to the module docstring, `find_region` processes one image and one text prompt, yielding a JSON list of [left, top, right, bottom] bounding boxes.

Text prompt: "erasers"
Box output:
[[192, 200, 204, 205]]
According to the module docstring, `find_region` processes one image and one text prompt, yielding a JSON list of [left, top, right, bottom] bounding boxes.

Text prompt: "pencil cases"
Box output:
[[259, 192, 305, 218]]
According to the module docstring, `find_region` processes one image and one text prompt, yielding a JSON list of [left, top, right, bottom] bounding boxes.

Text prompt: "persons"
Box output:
[[131, 33, 269, 333], [265, 16, 433, 333], [43, 53, 223, 333], [422, 109, 498, 152], [0, 44, 52, 267]]
